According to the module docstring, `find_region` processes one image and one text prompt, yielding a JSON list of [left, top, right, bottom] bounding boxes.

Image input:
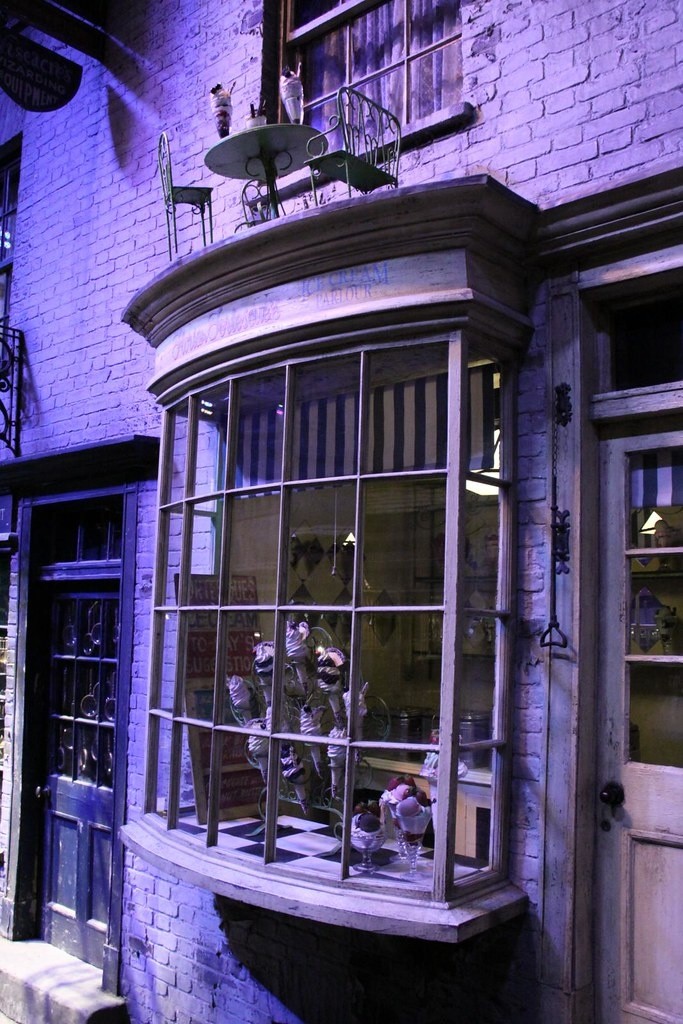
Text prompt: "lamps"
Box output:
[[639, 511, 664, 535], [466, 417, 501, 495]]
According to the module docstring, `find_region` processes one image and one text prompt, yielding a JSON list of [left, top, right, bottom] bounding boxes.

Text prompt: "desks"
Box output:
[[204, 123, 328, 230]]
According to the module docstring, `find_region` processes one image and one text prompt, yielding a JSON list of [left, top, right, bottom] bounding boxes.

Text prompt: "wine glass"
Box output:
[[350, 835, 385, 872], [388, 804, 410, 861], [396, 814, 431, 881]]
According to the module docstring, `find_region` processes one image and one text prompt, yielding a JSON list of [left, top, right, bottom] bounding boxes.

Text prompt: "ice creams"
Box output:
[[226, 621, 366, 815], [347, 774, 434, 851]]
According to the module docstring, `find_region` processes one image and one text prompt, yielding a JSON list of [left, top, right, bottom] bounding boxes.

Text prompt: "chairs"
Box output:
[[304, 87, 401, 204], [234, 179, 277, 233], [157, 132, 214, 261]]
